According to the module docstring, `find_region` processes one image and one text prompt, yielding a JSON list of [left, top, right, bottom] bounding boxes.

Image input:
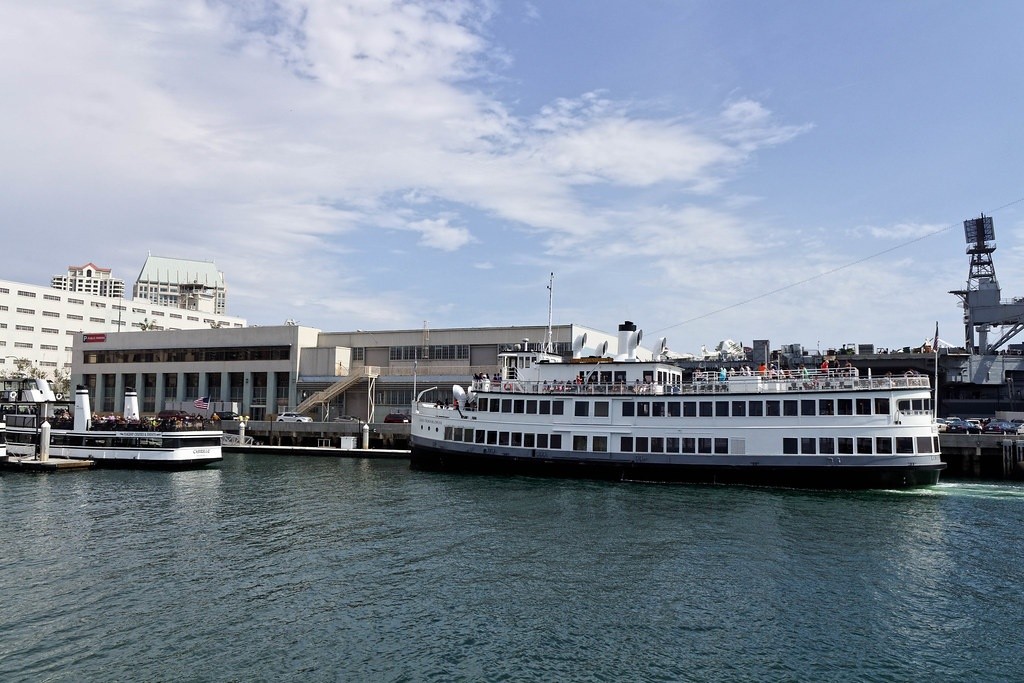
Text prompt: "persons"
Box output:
[[91, 412, 221, 432], [472, 359, 899, 393], [436, 398, 449, 409]]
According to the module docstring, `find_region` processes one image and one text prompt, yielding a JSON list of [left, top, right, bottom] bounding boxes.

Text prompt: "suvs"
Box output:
[[159, 410, 191, 419]]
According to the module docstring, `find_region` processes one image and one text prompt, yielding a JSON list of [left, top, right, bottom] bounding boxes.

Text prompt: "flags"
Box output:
[[194, 396, 209, 409]]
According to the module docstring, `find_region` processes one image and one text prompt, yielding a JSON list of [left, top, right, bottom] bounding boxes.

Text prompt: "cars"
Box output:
[[384, 413, 411, 423], [276, 412, 313, 422], [333, 416, 364, 423], [937, 417, 1024, 433], [212, 411, 239, 420]]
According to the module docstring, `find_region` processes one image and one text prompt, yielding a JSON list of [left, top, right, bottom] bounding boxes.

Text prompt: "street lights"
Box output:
[[5, 355, 21, 371]]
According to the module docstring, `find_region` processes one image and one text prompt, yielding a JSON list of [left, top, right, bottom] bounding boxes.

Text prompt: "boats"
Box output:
[[0, 377, 223, 469], [410, 273, 947, 488]]
